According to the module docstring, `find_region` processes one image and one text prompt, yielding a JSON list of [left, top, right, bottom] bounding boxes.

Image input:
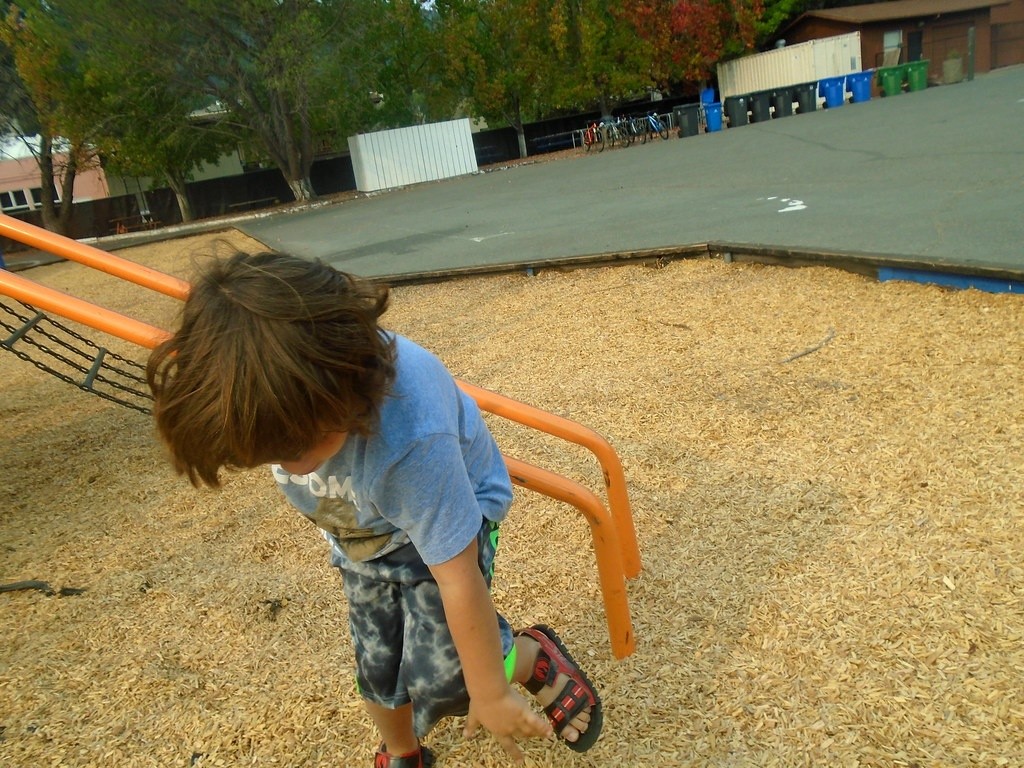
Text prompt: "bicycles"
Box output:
[[640, 109, 669, 142], [581, 120, 605, 154], [621, 112, 646, 144], [601, 117, 630, 149]]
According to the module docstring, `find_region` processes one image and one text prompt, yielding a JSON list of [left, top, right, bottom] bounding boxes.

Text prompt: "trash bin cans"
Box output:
[[877, 66, 902, 98], [747, 91, 770, 123], [702, 88, 722, 132], [672, 103, 700, 137], [793, 81, 817, 114], [819, 76, 844, 108], [770, 87, 792, 118], [901, 60, 930, 93], [846, 72, 872, 104], [723, 95, 747, 129]]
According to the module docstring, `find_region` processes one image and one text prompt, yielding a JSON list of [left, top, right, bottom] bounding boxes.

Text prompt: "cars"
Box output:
[[229, 190, 282, 212], [535, 129, 582, 155], [474, 145, 503, 166]]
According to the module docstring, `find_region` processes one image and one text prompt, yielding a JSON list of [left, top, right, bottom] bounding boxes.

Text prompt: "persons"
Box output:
[[145, 236, 605, 768]]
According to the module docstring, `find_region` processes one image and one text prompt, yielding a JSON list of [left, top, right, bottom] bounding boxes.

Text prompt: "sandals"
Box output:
[[376, 739, 433, 768], [515, 624, 604, 754]]
